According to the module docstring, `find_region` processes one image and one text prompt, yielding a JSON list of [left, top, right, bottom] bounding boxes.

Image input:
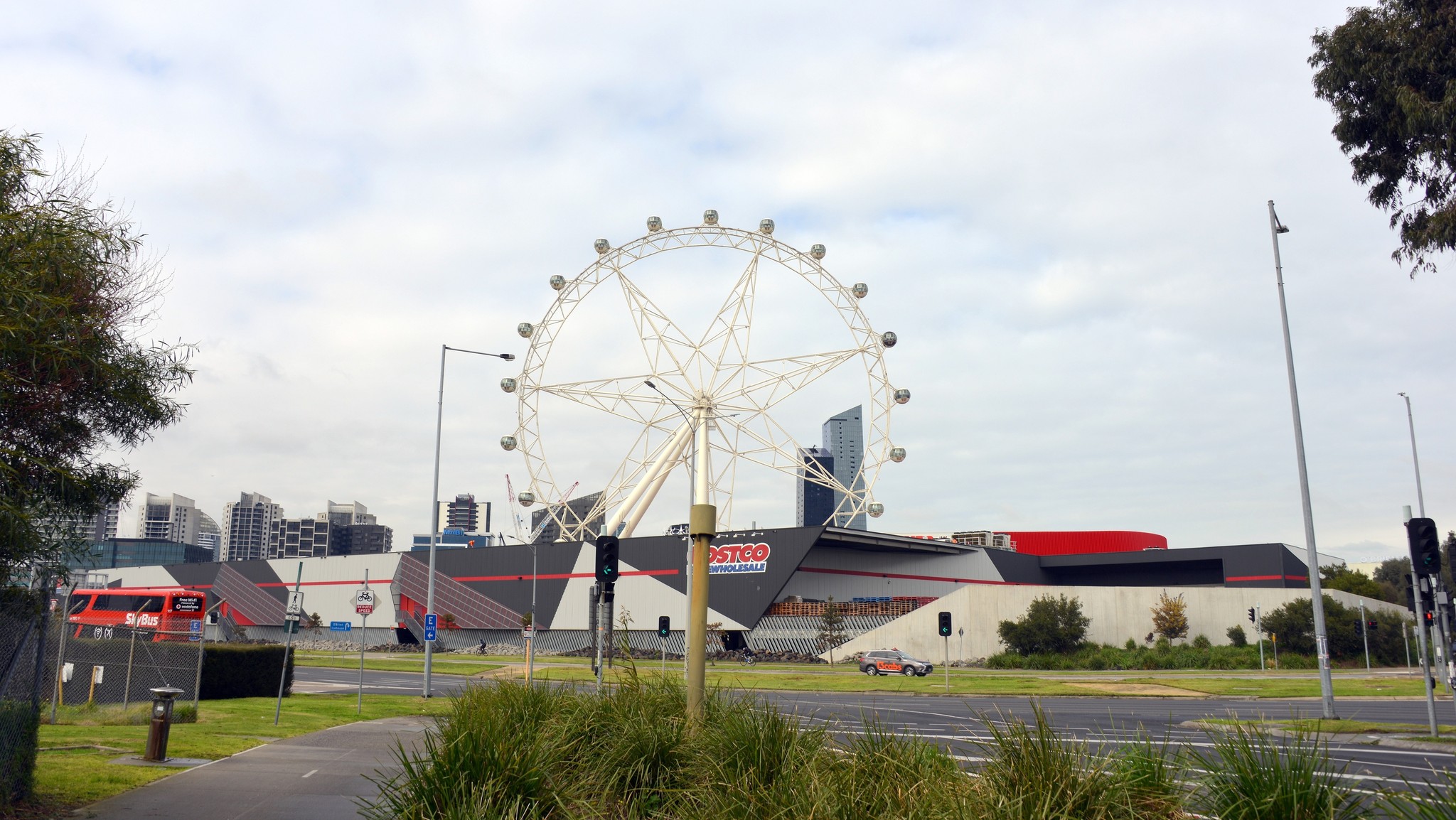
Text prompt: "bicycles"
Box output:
[[740, 654, 757, 667], [476, 646, 489, 657]]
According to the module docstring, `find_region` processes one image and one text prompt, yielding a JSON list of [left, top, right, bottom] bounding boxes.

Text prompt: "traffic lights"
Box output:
[[211, 612, 217, 623], [595, 536, 619, 582], [1248, 606, 1255, 624], [594, 581, 615, 603], [939, 611, 952, 636], [1408, 518, 1441, 574], [1448, 543, 1456, 583], [1354, 619, 1362, 634], [1404, 573, 1435, 612], [659, 616, 670, 637], [1368, 621, 1378, 630], [1423, 613, 1433, 626]]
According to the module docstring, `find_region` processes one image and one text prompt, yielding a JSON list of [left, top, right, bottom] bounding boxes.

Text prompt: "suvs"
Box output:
[[859, 650, 934, 677]]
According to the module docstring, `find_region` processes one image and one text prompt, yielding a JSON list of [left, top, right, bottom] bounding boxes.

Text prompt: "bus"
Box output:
[[66, 589, 206, 646]]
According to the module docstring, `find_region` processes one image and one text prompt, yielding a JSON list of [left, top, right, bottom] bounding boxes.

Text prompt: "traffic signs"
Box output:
[[424, 614, 437, 641]]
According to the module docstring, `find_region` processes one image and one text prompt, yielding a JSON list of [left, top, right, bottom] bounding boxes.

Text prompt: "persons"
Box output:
[[479, 639, 487, 654], [741, 647, 753, 662], [469, 540, 475, 548]]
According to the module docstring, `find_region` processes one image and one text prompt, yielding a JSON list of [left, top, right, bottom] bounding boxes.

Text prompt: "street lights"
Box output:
[[1267, 199, 1343, 723], [644, 380, 740, 684], [1397, 391, 1452, 696], [12, 555, 62, 594], [506, 535, 537, 690], [423, 345, 515, 698]]
